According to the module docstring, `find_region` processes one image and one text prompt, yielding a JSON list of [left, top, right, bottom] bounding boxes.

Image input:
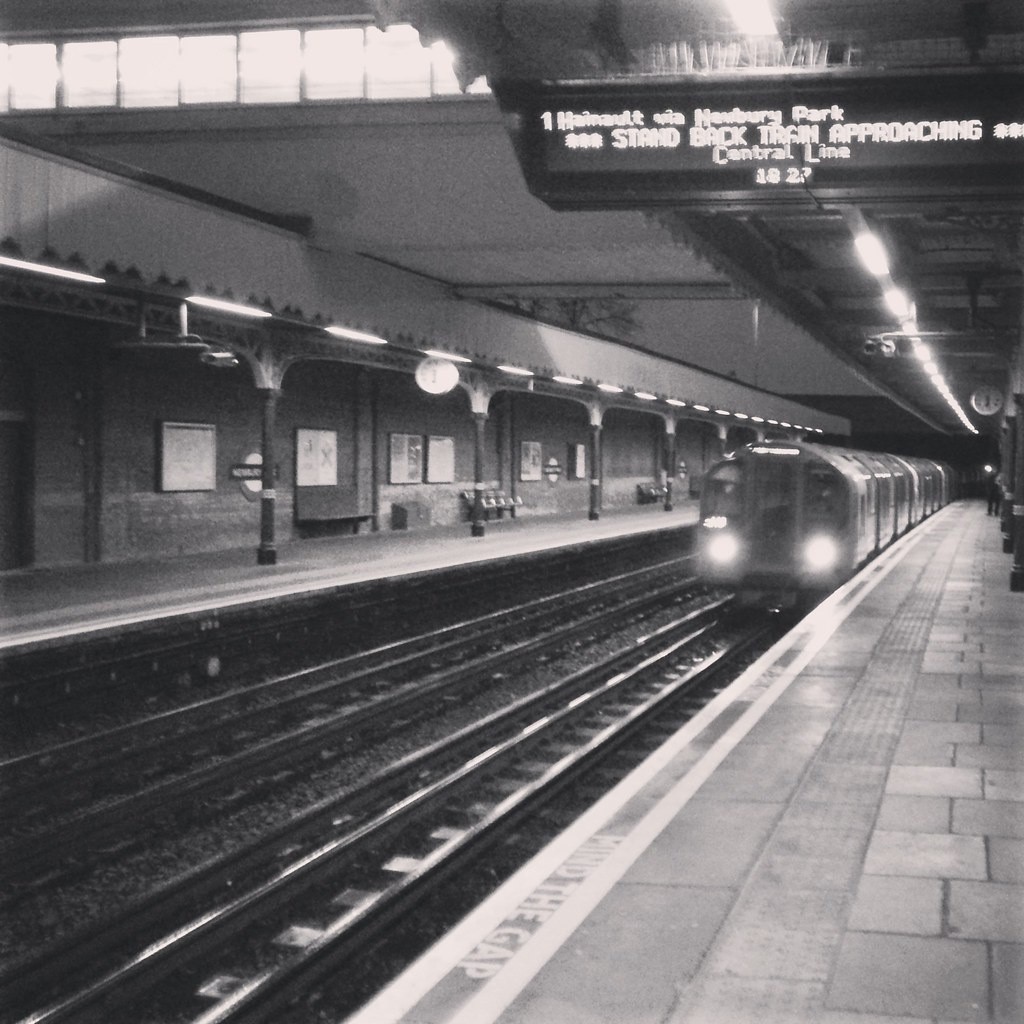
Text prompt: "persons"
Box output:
[[986, 467, 1001, 517]]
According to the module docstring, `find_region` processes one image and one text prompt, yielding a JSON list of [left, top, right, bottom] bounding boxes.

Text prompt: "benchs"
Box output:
[[461, 489, 522, 518], [638, 482, 667, 501], [296, 486, 374, 537]]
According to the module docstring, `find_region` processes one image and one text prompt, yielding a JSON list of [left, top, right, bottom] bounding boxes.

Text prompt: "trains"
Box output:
[[696, 438, 959, 611]]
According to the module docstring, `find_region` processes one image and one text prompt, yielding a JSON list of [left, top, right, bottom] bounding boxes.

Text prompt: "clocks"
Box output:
[[971, 383, 1003, 415]]
